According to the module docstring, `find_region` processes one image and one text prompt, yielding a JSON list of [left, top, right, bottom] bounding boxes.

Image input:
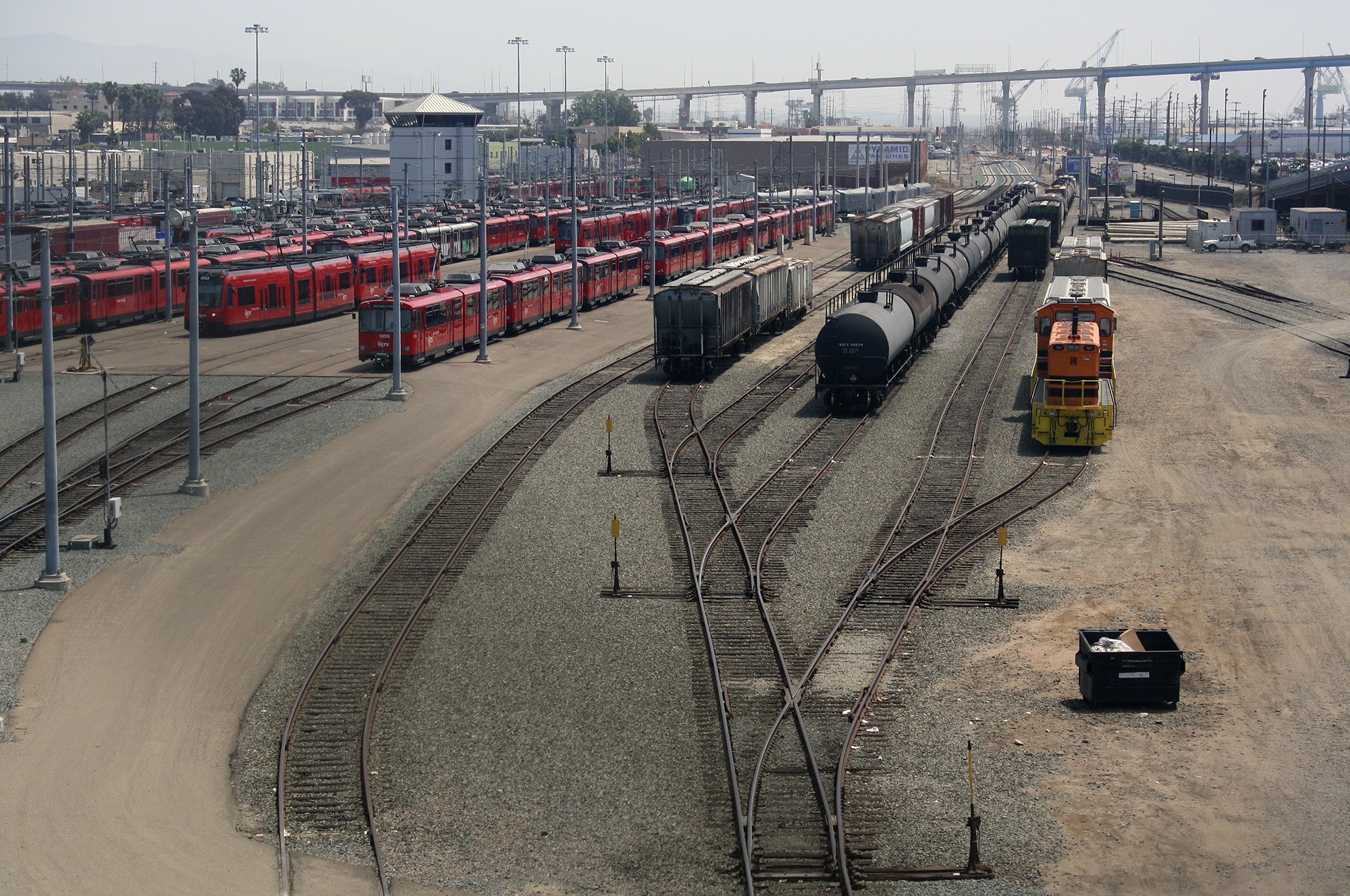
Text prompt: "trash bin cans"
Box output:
[[1130, 201, 1139, 217], [1074, 628, 1186, 708]]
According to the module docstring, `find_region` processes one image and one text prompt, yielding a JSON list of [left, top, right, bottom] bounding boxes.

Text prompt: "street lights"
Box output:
[[1186, 175, 1193, 185], [556, 45, 629, 204], [284, 153, 300, 188], [1133, 170, 1154, 201], [310, 152, 319, 190], [168, 206, 210, 498], [563, 128, 584, 333], [507, 36, 529, 199], [1205, 175, 1213, 187], [1169, 174, 1175, 187], [245, 23, 269, 159], [1261, 88, 1267, 163], [433, 132, 442, 201], [300, 130, 308, 255], [1307, 86, 1313, 210]]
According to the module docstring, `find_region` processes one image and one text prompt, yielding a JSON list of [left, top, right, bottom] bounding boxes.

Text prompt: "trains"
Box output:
[[808, 179, 1038, 413], [0, 174, 917, 345], [850, 189, 955, 268], [1031, 234, 1120, 447], [653, 253, 812, 373], [1006, 174, 1077, 279], [356, 199, 838, 371]]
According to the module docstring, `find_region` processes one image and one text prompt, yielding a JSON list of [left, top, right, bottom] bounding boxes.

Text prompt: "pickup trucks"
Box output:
[[1202, 234, 1258, 253]]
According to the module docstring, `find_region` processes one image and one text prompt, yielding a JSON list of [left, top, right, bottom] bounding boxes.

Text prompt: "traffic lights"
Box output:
[[904, 179, 908, 188]]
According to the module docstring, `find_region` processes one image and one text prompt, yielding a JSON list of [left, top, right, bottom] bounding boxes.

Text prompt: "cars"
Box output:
[[928, 140, 981, 159]]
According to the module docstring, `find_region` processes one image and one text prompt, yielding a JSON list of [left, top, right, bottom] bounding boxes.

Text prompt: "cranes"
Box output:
[[991, 60, 1050, 133], [1063, 28, 1126, 122]]
